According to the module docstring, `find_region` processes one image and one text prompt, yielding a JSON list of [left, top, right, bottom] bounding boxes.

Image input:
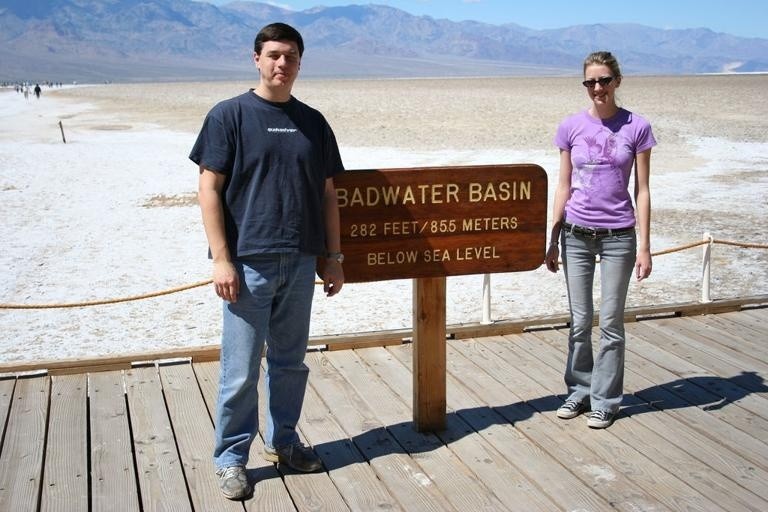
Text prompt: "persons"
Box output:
[[545, 51, 656, 430], [188, 22, 345, 500], [15, 82, 42, 100]]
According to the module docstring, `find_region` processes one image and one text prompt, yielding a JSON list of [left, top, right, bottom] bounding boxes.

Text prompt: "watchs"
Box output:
[[325, 251, 345, 265]]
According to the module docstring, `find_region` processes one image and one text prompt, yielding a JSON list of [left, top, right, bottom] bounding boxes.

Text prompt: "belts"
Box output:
[[561, 222, 635, 238]]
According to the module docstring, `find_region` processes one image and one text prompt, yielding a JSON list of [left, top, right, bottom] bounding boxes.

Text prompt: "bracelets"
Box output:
[[548, 240, 560, 247]]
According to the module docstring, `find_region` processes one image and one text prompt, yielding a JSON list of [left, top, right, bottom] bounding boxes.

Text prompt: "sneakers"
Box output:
[[215, 462, 253, 501], [587, 410, 617, 428], [556, 398, 585, 420], [262, 439, 324, 473]]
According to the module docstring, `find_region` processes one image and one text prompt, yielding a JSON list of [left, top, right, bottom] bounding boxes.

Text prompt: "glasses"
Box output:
[[581, 75, 619, 88]]
